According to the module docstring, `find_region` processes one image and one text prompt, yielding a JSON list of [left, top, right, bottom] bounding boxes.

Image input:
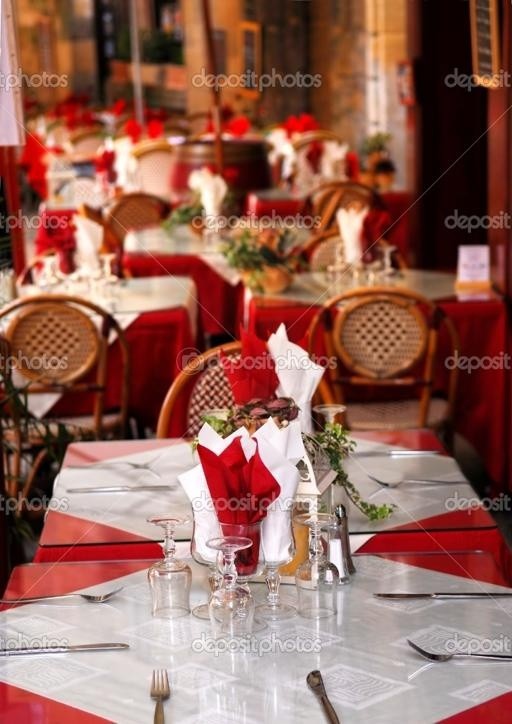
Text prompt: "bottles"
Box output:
[[336, 502, 357, 578]]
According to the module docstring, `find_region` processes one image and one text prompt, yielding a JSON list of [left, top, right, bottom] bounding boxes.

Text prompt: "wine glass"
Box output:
[[312, 403, 347, 425], [146, 512, 339, 638], [35, 253, 121, 313], [326, 240, 397, 290]]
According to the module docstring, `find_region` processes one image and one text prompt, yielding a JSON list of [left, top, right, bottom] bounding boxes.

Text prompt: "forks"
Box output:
[[368, 475, 470, 489], [150, 668, 171, 724], [406, 638, 512, 663], [0, 586, 123, 602], [67, 453, 159, 468]]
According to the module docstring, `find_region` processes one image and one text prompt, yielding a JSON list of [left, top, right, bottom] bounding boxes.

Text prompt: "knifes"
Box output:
[[356, 449, 439, 457], [375, 592, 512, 600], [306, 670, 340, 724], [66, 485, 173, 493], [0, 643, 131, 655]]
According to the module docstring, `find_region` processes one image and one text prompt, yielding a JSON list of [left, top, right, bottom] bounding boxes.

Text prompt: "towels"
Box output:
[[177, 413, 300, 574]]
